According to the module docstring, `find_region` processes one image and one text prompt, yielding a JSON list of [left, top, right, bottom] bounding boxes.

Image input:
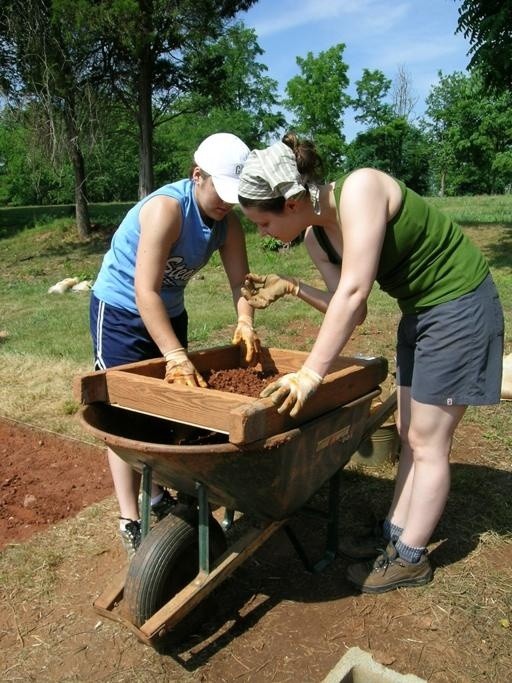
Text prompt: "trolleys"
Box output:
[[74, 389, 398, 646]]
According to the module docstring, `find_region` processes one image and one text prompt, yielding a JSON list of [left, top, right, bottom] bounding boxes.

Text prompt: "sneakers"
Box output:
[[345, 535, 433, 594], [341, 526, 390, 557], [138, 491, 177, 521], [118, 517, 142, 563]]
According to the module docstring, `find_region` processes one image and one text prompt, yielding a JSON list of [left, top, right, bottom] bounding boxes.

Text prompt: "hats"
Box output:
[[194, 133, 250, 203]]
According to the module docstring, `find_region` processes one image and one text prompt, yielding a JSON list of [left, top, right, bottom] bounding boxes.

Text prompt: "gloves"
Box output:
[[162, 348, 209, 387], [240, 273, 300, 309], [232, 315, 262, 362], [259, 365, 323, 417]]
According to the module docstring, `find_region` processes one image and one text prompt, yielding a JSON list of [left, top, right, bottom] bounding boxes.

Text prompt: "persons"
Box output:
[[88, 132, 264, 556], [237, 133, 504, 592]]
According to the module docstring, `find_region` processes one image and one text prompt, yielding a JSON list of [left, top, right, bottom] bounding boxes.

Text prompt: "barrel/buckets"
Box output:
[[353, 423, 396, 466]]
